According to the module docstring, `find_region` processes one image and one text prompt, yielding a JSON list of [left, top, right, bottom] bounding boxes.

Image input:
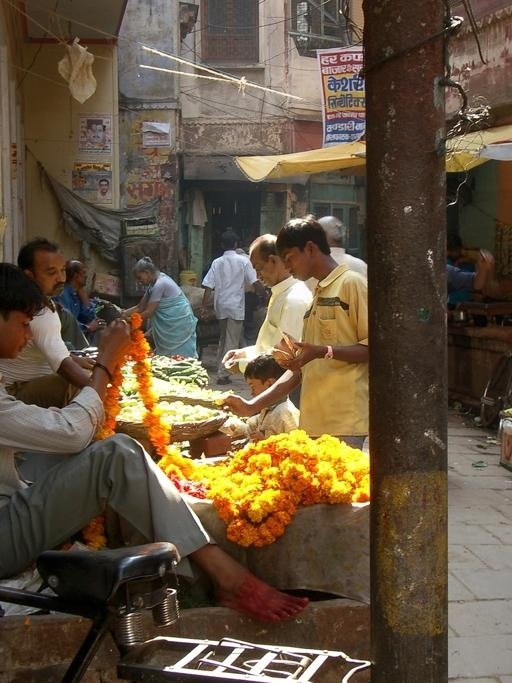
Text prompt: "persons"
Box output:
[[216, 232, 314, 410], [97, 179, 113, 204], [114, 254, 200, 358], [1, 262, 312, 627], [3, 234, 96, 409], [240, 353, 301, 443], [219, 215, 369, 451], [315, 214, 368, 281], [193, 232, 268, 384], [51, 257, 108, 333], [447, 234, 496, 305]]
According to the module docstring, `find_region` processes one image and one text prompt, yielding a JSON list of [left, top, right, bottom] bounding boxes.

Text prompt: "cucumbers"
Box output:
[[152, 359, 210, 389]]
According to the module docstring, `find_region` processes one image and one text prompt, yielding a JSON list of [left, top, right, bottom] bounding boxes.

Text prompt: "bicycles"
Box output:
[[479, 350, 511, 428], [0, 540, 374, 682]]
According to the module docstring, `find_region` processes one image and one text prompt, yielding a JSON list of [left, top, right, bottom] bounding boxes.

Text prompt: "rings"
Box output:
[[99, 322, 102, 324]]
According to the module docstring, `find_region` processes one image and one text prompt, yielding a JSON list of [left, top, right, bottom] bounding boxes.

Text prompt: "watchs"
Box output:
[[323, 344, 334, 360]]
